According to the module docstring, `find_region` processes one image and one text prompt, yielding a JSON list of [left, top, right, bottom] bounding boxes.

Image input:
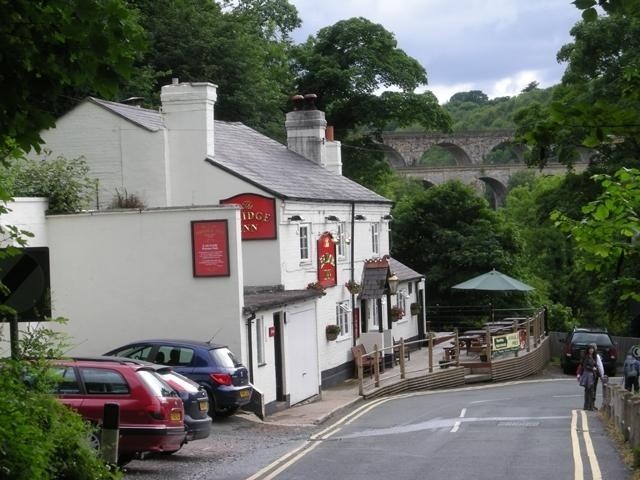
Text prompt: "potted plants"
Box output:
[[326, 324, 340, 340], [391, 307, 402, 321]]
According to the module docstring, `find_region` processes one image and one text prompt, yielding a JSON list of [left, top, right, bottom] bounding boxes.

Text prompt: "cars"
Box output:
[[558, 324, 619, 376], [21, 327, 254, 459]]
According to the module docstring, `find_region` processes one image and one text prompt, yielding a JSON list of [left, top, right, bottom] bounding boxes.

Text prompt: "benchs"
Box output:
[[351, 343, 383, 379]]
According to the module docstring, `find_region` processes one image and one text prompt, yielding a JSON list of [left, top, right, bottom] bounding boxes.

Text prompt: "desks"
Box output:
[[443, 321, 519, 360]]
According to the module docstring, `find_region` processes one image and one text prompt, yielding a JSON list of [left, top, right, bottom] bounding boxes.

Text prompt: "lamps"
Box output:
[[387, 272, 401, 295]]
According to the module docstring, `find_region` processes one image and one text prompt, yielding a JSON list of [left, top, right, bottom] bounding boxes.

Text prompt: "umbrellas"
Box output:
[[451, 267, 537, 327]]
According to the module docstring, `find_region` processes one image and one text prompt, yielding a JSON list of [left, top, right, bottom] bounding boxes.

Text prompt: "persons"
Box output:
[[624, 354, 640, 395], [580, 346, 598, 411], [576, 343, 604, 411]]
